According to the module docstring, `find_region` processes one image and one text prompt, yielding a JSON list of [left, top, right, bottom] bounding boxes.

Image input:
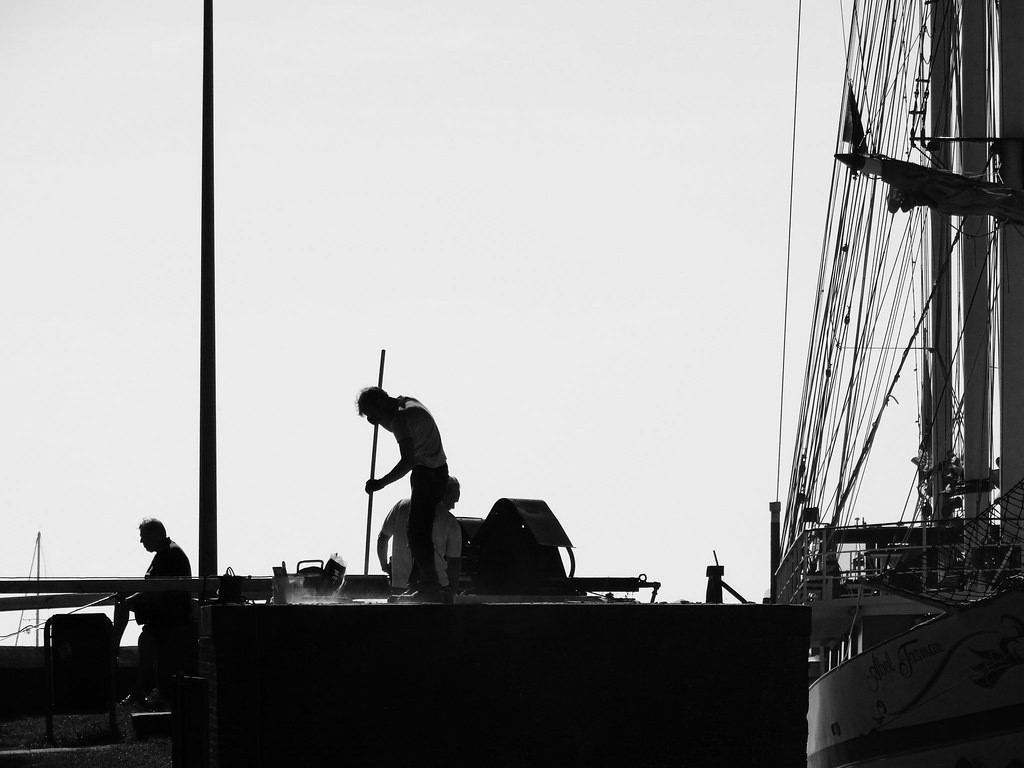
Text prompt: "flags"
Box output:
[[842, 85, 867, 154]]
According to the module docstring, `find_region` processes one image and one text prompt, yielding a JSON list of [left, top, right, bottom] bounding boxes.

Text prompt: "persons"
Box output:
[[358, 388, 448, 604], [116, 520, 194, 706], [378, 477, 462, 595]]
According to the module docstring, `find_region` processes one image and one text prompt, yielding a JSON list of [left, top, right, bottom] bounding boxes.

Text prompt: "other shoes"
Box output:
[[387, 589, 411, 603], [397, 591, 430, 602]]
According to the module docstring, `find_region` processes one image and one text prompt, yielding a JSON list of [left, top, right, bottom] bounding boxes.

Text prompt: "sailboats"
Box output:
[[763, 0, 1024, 768]]
